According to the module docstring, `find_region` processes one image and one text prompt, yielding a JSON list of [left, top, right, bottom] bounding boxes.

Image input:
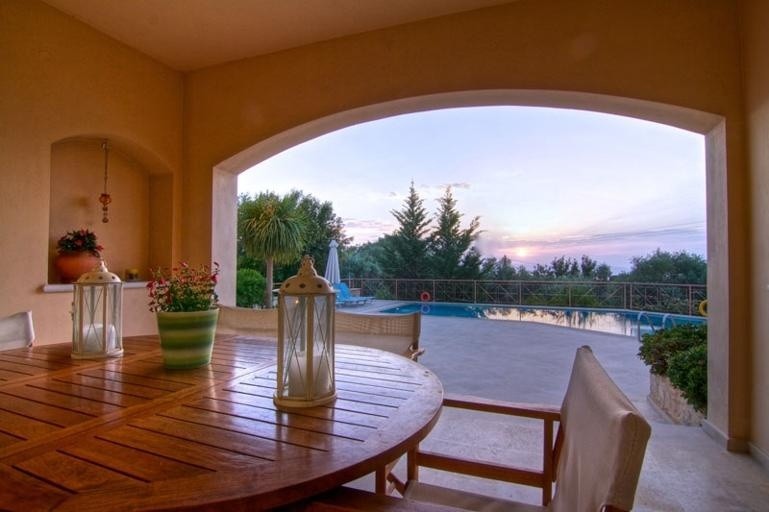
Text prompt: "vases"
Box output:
[[52, 251, 99, 285], [154, 306, 220, 369]]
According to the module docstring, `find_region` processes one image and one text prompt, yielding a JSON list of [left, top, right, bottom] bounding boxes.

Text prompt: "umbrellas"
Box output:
[[322, 238, 343, 285]]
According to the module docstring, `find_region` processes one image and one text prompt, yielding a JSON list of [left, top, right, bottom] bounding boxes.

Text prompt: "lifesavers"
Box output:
[[422, 305, 430, 315], [699, 300, 708, 316], [421, 292, 430, 302]]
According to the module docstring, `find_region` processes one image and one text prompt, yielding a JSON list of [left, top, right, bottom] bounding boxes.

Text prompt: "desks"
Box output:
[[1, 334, 446, 512]]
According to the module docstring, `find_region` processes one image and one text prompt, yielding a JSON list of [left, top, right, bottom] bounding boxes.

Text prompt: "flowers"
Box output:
[[55, 225, 104, 254], [141, 257, 223, 314]]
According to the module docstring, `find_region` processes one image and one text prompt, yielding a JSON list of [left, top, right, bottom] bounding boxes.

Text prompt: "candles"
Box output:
[[82, 323, 115, 350]]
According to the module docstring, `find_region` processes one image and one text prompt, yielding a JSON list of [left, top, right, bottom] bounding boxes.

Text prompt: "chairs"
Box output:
[[0, 309, 36, 351], [329, 281, 376, 308], [311, 345, 652, 512]]
